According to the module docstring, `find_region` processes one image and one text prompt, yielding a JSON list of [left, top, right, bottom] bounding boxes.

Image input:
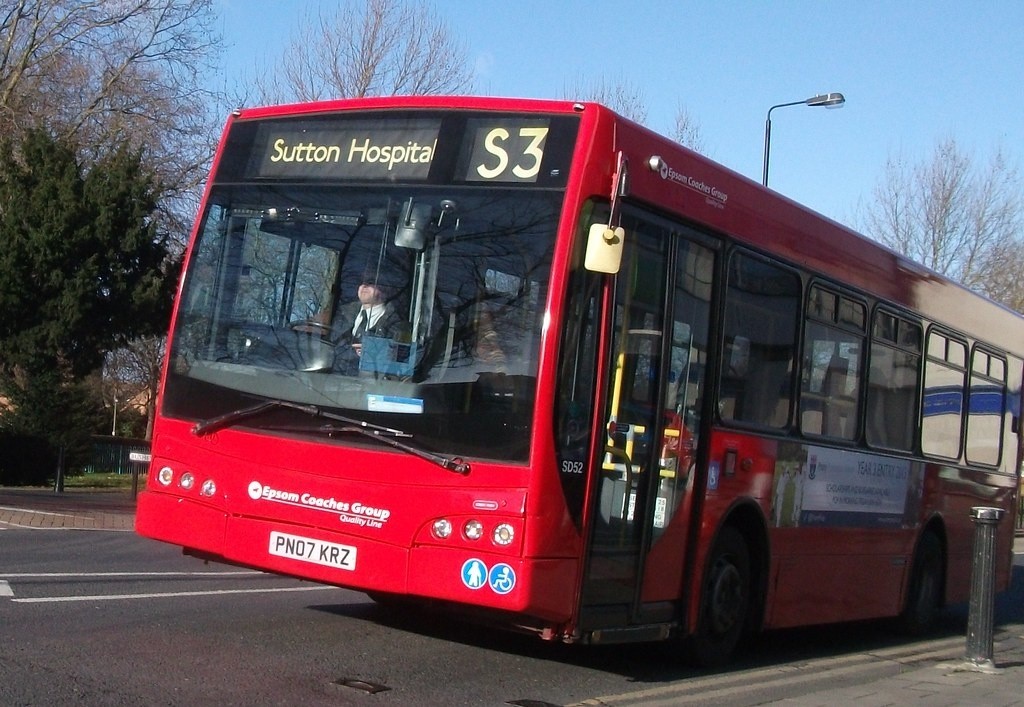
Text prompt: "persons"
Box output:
[[329, 270, 402, 376]]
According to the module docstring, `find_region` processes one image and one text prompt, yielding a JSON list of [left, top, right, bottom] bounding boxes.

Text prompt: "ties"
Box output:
[[354, 309, 367, 340]]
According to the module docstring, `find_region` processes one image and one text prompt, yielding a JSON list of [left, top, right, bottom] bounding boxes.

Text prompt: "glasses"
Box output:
[[360, 279, 379, 286]]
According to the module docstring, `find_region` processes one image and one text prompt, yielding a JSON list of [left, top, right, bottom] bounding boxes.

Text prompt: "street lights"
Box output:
[[762, 92, 845, 188]]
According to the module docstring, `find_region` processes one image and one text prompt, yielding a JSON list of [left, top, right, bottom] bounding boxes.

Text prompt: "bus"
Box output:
[[135, 91, 1024, 675]]
